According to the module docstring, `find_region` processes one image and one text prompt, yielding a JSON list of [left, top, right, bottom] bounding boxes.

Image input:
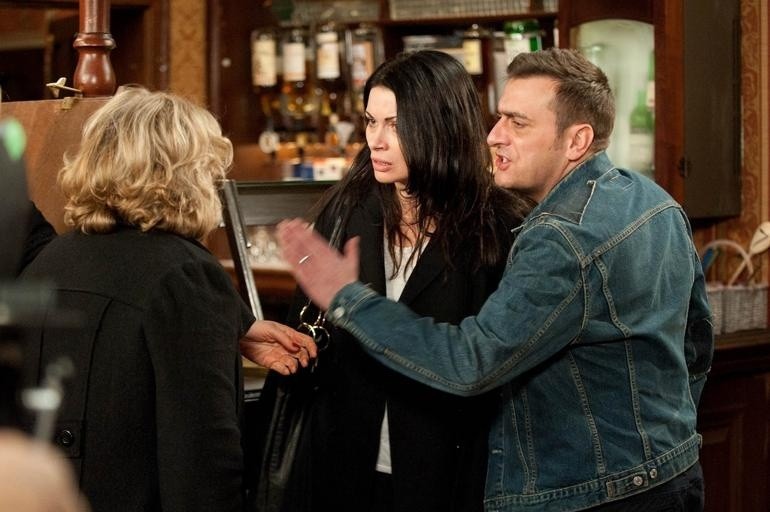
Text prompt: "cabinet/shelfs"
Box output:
[[171, 1, 739, 216]]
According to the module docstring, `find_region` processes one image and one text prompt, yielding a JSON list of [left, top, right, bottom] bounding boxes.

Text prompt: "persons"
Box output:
[[282, 51, 533, 512], [277, 49, 716, 512], [0, 83, 318, 512]]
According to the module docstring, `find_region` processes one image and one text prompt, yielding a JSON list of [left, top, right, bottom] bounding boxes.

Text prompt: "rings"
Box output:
[[296, 253, 312, 266]]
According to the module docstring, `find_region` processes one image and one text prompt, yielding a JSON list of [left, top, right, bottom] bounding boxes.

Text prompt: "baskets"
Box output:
[[704, 284, 724, 337], [698, 239, 770, 334]]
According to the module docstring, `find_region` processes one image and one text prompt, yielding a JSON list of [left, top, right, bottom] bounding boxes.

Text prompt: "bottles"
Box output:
[[250, 25, 280, 160], [348, 27, 385, 136], [505, 17, 542, 63], [312, 28, 350, 156], [280, 35, 313, 158], [463, 28, 493, 109], [629, 48, 655, 176]]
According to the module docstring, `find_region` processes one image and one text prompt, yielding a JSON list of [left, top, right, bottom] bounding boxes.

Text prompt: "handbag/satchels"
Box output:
[[253, 193, 357, 512]]
